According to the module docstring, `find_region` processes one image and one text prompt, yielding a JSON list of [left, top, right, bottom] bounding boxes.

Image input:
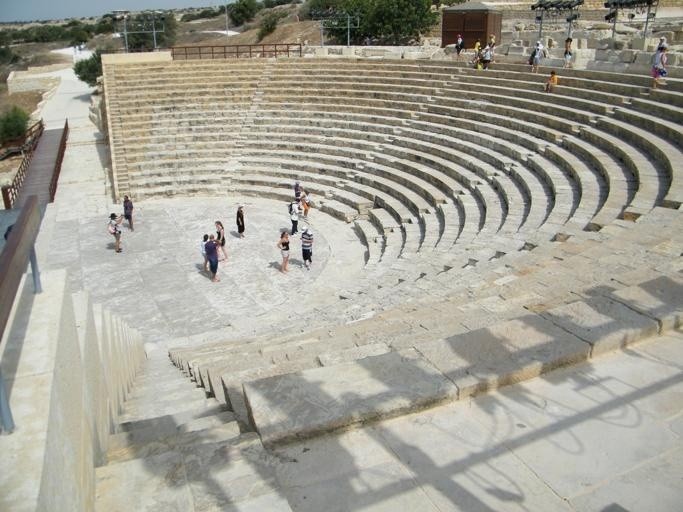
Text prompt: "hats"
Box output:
[[108, 214, 118, 218], [300, 225, 308, 233]]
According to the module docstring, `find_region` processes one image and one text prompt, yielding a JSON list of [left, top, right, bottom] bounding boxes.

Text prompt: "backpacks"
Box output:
[[107, 221, 116, 235], [288, 203, 293, 214]]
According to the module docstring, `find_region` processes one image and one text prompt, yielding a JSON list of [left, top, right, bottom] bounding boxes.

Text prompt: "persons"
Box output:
[[294, 183, 303, 211], [455, 35, 463, 55], [289, 198, 302, 237], [530, 41, 543, 74], [300, 189, 310, 218], [655, 36, 667, 51], [204, 234, 222, 283], [299, 226, 313, 269], [277, 231, 290, 274], [236, 205, 244, 238], [213, 221, 229, 261], [122, 195, 134, 232], [488, 34, 495, 64], [479, 46, 491, 70], [563, 38, 572, 68], [650, 44, 667, 90], [544, 71, 557, 93], [473, 39, 481, 64], [200, 234, 209, 274], [109, 213, 125, 253]]
